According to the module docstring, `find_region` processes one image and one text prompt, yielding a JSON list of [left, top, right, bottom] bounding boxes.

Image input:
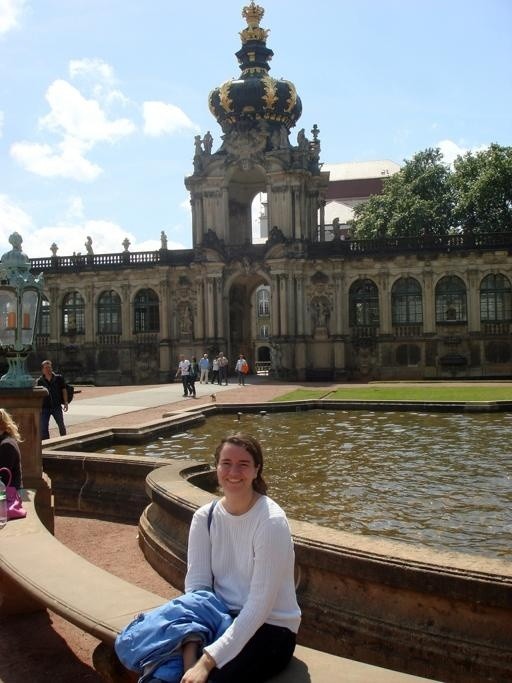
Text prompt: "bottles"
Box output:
[[0, 476, 7, 525]]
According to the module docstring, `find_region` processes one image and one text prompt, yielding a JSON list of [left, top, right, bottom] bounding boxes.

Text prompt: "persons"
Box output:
[[0, 408, 26, 490], [37, 360, 68, 440], [148, 434, 303, 683], [175, 352, 249, 398]]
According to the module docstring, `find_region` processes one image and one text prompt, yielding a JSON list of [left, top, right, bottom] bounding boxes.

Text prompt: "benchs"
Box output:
[[0, 487, 442, 683]]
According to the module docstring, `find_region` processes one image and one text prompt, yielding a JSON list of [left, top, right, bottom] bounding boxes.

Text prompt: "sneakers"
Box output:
[[200, 381, 228, 386], [239, 383, 244, 386], [183, 389, 197, 397]]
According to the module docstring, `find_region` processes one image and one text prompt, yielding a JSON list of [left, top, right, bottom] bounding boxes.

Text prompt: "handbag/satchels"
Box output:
[[0, 486, 27, 520], [61, 384, 74, 405], [241, 363, 248, 374]]
